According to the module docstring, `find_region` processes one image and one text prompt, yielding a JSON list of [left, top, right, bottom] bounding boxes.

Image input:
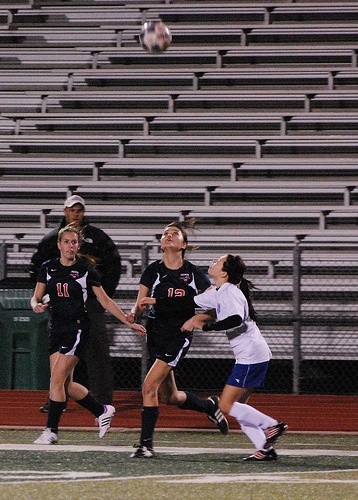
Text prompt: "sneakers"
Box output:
[[205, 395, 230, 436], [32, 427, 60, 445], [128, 443, 158, 458], [242, 445, 278, 462], [38, 403, 66, 413], [261, 419, 289, 450], [94, 404, 116, 439]]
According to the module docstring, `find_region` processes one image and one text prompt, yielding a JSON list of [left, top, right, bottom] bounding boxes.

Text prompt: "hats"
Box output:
[[63, 194, 86, 209]]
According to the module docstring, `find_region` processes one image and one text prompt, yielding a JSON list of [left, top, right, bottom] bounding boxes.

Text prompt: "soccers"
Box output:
[[138, 21, 173, 53]]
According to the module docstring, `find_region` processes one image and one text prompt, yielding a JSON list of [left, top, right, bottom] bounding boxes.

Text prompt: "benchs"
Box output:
[[0, 0, 358, 296]]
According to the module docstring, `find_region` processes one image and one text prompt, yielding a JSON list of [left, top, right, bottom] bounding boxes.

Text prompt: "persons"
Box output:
[[30, 222, 146, 445], [126, 222, 229, 458], [138, 255, 288, 461], [30, 195, 121, 413]]
